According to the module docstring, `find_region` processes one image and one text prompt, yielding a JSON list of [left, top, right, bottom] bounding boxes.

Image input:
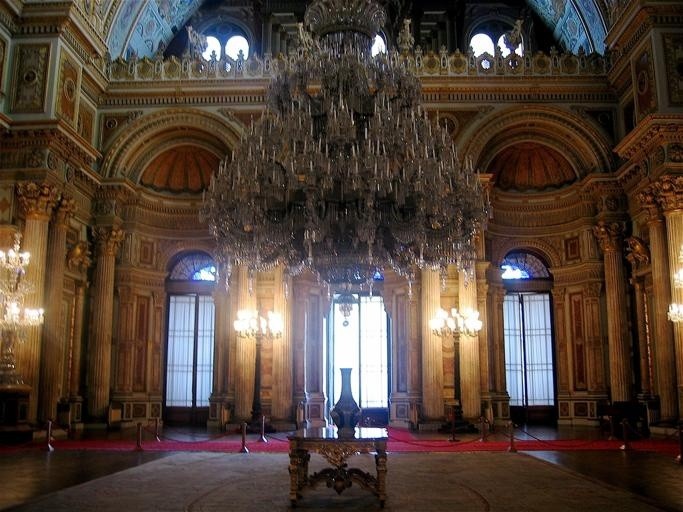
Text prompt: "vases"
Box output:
[[330, 368, 362, 428]]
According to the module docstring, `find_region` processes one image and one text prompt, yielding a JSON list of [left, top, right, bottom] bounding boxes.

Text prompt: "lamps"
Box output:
[[196, 1, 495, 323], [0, 221, 46, 384]]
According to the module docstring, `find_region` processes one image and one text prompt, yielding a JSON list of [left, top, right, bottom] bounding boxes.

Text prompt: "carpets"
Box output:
[[0, 451, 683, 511]]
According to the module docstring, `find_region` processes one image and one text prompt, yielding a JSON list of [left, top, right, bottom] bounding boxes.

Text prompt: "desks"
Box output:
[[287, 427, 389, 510]]
